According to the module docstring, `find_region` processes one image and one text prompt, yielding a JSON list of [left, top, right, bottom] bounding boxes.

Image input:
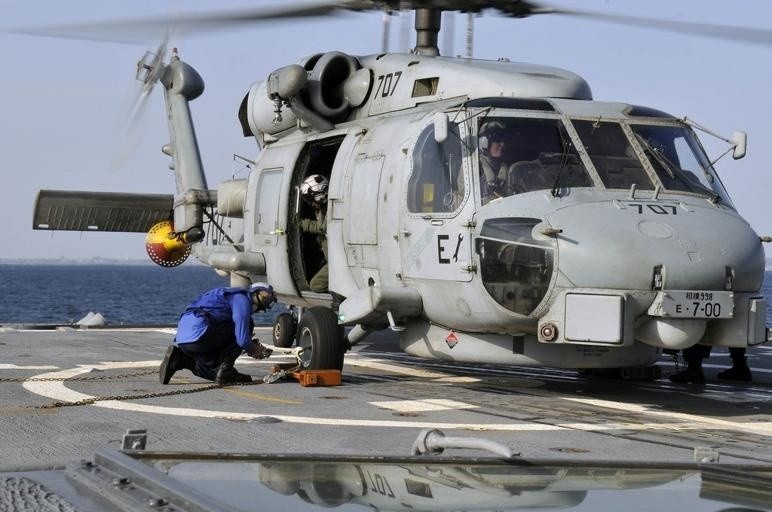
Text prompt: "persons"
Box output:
[[456, 120, 512, 202], [292, 173, 330, 294], [159, 282, 278, 387], [667, 343, 755, 384]]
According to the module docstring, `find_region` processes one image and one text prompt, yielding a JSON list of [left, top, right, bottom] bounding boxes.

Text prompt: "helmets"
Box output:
[[478, 120, 507, 155], [254, 290, 278, 313], [300, 174, 328, 207]]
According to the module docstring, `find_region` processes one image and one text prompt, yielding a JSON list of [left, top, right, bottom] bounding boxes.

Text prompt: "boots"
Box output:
[[160, 345, 190, 384], [669, 359, 705, 383], [717, 355, 752, 382], [216, 363, 252, 384]]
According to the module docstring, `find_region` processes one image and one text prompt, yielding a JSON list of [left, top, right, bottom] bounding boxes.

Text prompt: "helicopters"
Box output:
[[0, 0, 772, 370]]
